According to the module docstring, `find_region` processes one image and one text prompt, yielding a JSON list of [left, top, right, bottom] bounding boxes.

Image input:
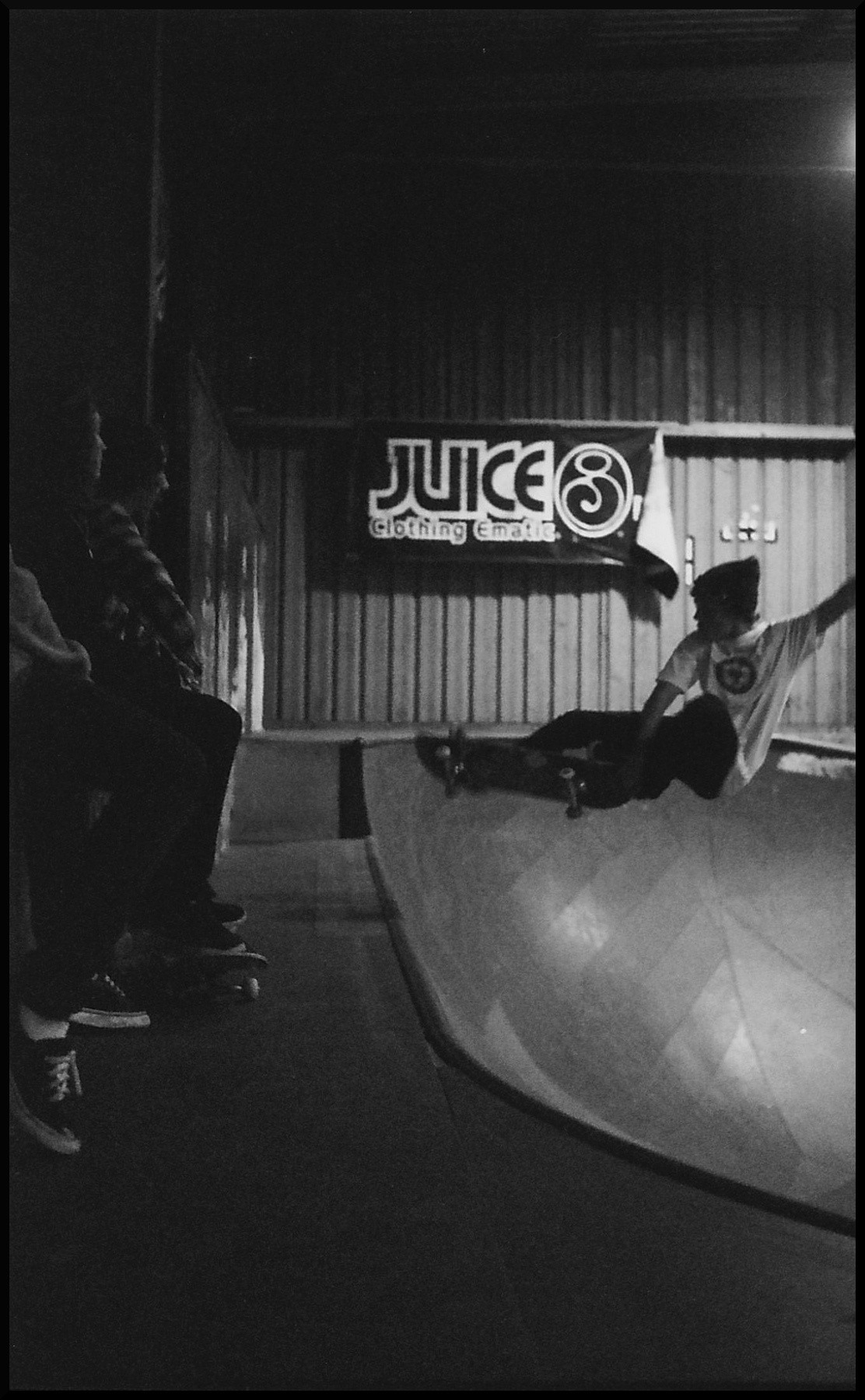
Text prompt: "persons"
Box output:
[[9, 395, 269, 1157], [415, 557, 856, 809]]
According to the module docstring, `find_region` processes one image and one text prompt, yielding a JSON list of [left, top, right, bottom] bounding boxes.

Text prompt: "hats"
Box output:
[[693, 555, 761, 615]]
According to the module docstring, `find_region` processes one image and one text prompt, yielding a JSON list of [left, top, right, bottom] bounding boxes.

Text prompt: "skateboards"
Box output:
[[183, 944, 268, 1001], [414, 736, 629, 819]]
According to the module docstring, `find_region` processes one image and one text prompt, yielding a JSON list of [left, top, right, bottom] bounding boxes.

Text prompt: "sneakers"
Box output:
[[9, 1007, 83, 1156], [69, 970, 151, 1027]]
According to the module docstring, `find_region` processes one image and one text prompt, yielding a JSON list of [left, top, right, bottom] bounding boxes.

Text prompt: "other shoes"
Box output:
[[191, 895, 249, 925], [131, 899, 245, 958]]
